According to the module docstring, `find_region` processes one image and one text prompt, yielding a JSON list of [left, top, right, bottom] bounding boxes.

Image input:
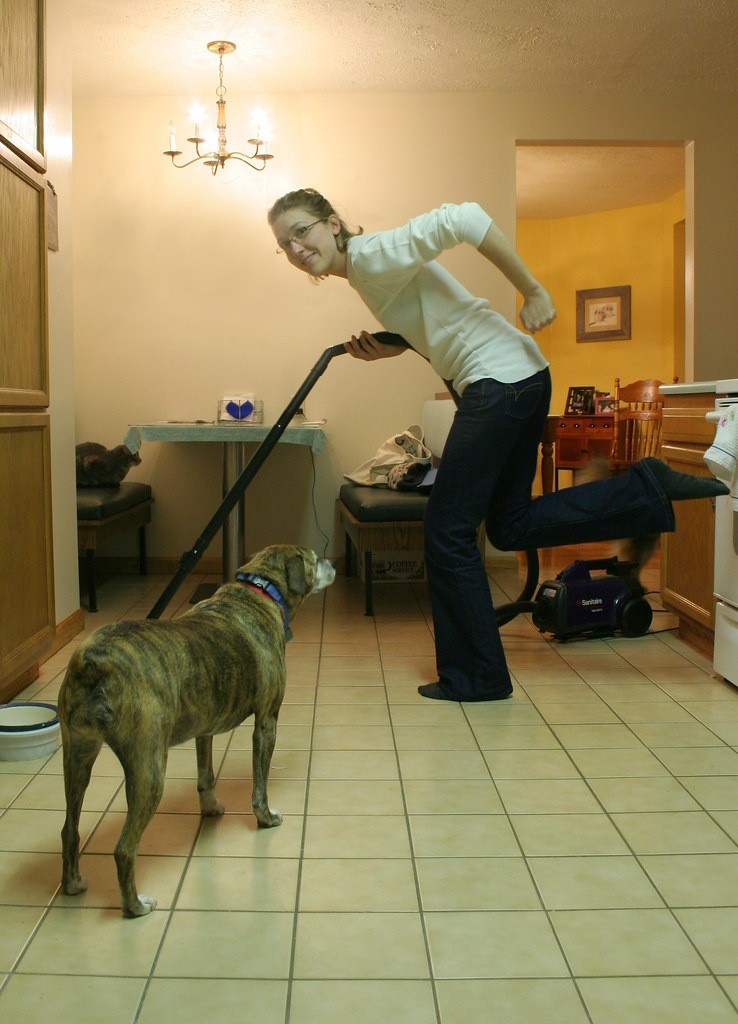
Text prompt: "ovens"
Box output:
[[712, 397, 738, 689]]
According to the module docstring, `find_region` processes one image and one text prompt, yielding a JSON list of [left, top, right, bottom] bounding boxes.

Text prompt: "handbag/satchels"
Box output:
[[343, 424, 433, 489]]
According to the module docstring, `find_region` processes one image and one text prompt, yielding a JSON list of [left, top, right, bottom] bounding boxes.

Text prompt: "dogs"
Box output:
[[57, 545, 336, 919]]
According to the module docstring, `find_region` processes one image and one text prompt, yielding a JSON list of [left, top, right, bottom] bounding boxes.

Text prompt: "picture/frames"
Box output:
[[575, 285, 631, 344], [565, 385, 595, 415], [596, 398, 614, 414]]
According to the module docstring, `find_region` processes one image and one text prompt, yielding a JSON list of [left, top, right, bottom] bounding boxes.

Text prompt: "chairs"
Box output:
[[77, 482, 154, 612], [610, 376, 678, 470], [336, 391, 484, 617]]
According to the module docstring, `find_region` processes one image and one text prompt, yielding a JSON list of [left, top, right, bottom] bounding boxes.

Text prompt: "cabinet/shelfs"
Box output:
[[661, 394, 738, 688], [0, 1, 56, 689]]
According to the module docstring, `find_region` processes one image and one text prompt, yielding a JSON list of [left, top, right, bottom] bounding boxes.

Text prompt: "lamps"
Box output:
[[163, 42, 275, 176]]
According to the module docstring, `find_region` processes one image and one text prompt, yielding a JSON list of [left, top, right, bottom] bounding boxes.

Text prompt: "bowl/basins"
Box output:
[[0, 702, 61, 762]]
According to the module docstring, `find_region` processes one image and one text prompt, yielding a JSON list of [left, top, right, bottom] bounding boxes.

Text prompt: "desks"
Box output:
[[124, 420, 326, 605], [541, 413, 632, 495]]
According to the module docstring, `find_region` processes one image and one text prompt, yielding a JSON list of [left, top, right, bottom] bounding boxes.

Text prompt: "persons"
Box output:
[[267, 187, 731, 703]]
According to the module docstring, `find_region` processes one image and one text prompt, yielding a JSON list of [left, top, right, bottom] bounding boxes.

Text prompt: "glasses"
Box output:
[[275, 217, 328, 256]]
